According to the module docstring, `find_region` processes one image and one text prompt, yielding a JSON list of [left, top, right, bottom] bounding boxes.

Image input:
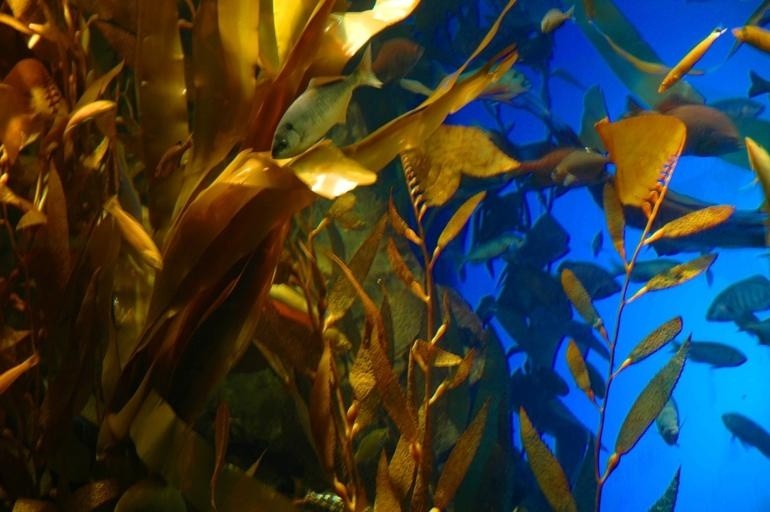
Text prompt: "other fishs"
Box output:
[[456, 0, 770, 457]]
